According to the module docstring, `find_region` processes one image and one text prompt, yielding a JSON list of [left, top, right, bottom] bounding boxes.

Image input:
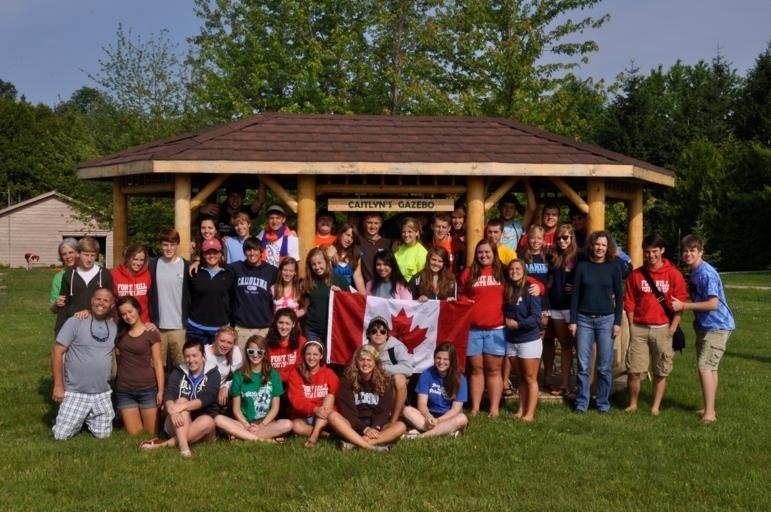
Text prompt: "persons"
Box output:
[[24, 252, 41, 273], [46, 179, 735, 459]]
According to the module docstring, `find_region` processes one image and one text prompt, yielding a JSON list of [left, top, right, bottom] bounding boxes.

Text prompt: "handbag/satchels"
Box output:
[[673, 324, 685, 355]]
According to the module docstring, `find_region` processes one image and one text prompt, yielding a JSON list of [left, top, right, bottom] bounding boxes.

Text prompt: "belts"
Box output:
[[583, 313, 612, 318]]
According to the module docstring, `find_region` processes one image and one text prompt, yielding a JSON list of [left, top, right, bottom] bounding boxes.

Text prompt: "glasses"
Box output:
[[90, 319, 110, 342], [246, 348, 265, 357], [557, 236, 569, 241], [371, 329, 387, 335]]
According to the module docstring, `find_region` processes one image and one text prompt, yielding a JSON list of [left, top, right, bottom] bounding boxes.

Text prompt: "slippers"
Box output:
[[179, 446, 192, 459], [541, 383, 571, 396], [273, 437, 286, 446], [139, 437, 160, 450]]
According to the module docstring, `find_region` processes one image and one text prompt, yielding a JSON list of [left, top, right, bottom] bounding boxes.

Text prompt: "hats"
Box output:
[[265, 205, 285, 214], [368, 316, 389, 327], [200, 237, 223, 253]]
